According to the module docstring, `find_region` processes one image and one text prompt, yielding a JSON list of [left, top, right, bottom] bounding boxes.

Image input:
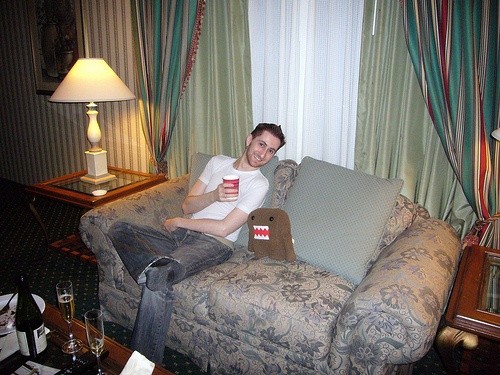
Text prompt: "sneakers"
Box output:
[[146, 258, 187, 291]]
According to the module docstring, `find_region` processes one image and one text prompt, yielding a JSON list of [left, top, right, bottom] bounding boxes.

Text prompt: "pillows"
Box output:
[[190, 151, 279, 247], [282, 154, 405, 287]]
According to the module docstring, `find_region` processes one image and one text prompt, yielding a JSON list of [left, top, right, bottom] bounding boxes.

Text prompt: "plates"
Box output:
[[92, 190, 108, 196], [0, 293, 45, 336]]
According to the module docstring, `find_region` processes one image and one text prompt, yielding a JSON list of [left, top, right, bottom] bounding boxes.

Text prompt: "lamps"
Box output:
[[47, 57, 137, 186]]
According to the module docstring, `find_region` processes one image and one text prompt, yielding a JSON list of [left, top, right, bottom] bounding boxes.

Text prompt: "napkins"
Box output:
[[0, 327, 51, 363], [118, 350, 157, 375]]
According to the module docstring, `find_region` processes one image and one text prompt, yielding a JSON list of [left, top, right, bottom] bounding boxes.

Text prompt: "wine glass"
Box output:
[[56, 281, 83, 353], [85, 309, 107, 375]]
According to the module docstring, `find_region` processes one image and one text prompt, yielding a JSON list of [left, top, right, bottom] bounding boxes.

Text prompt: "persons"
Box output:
[[110, 123, 286, 375]]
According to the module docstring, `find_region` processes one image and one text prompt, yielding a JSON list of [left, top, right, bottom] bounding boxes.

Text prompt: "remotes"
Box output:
[[54, 349, 109, 375]]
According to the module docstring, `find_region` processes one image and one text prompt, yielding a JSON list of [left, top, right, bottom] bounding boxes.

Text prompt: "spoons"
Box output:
[[0, 291, 18, 315]]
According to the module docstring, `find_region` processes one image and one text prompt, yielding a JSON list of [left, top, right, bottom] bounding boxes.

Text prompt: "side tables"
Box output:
[[431, 243, 500, 375], [25, 164, 170, 269]]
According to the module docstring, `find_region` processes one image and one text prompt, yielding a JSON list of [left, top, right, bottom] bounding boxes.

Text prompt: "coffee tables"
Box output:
[[0, 303, 176, 375]]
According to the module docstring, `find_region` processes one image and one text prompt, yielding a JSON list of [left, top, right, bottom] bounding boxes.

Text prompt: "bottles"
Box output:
[[15, 275, 49, 364]]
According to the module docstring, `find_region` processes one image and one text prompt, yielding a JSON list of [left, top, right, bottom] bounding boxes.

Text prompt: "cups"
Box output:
[[222, 175, 241, 200]]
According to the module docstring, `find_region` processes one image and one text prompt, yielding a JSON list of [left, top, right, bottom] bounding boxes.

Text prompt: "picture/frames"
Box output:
[[27, 0, 87, 96]]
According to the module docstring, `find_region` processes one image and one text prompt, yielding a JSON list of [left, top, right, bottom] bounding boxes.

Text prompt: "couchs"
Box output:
[[78, 175, 463, 375]]
